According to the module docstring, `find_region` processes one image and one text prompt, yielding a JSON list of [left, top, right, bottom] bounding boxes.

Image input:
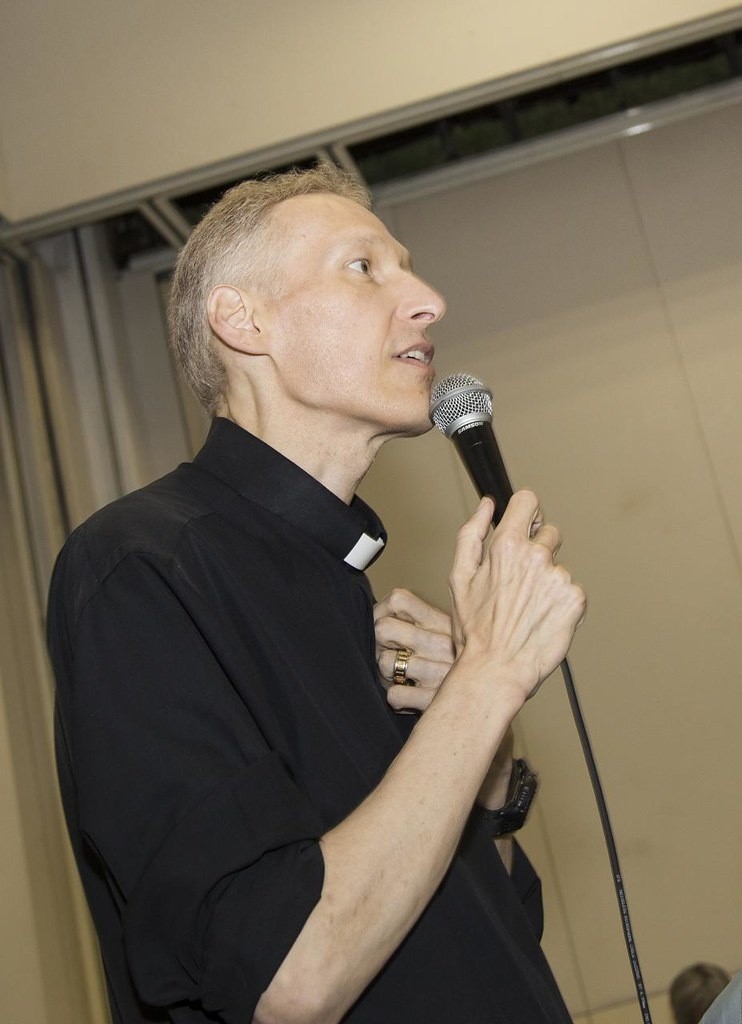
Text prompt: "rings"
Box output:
[[394, 650, 412, 685]]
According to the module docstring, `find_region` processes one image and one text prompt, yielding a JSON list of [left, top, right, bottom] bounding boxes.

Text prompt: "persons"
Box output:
[[671, 964, 730, 1024], [47, 171, 589, 1024]]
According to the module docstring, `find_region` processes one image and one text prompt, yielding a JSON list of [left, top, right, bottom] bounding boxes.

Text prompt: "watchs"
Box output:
[[478, 758, 538, 834]]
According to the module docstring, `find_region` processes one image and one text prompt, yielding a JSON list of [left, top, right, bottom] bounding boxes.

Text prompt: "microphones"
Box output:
[[429, 372, 513, 531]]
